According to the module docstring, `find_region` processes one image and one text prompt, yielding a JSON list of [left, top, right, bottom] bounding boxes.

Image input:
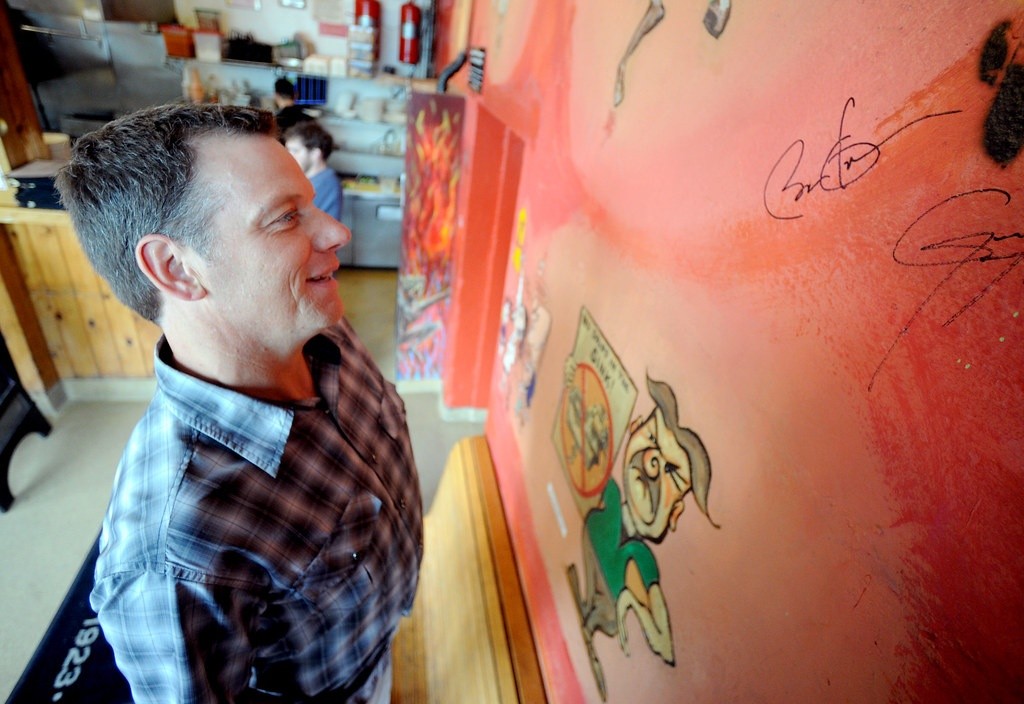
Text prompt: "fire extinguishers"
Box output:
[[400, 0, 421, 64], [356, 0, 380, 61]]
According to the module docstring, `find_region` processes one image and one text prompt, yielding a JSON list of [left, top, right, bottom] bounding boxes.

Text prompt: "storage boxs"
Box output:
[[162, 24, 193, 58], [192, 28, 224, 62]]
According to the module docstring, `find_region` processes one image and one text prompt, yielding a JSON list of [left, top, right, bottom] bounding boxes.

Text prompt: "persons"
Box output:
[[55, 102, 423, 704], [272, 75, 317, 147], [284, 122, 342, 220]]
